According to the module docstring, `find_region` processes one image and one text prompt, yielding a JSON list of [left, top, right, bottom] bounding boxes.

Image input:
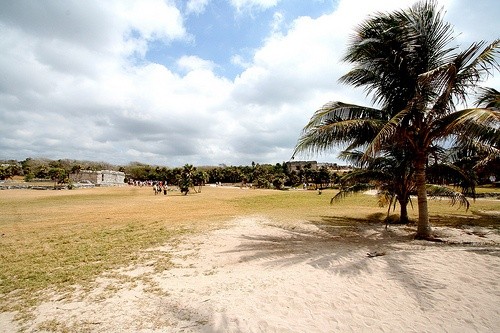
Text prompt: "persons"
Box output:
[[128, 178, 169, 195]]
